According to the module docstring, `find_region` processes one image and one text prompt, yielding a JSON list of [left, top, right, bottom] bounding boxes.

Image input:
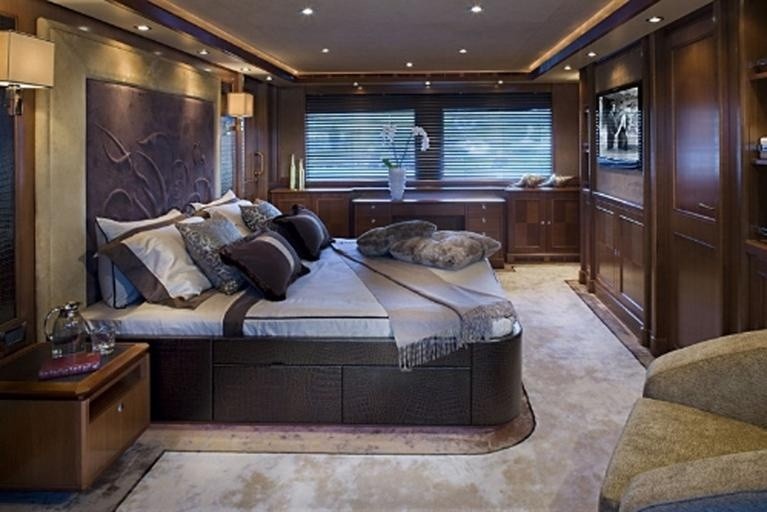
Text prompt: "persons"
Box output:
[[605, 101, 618, 150], [613, 98, 628, 151]]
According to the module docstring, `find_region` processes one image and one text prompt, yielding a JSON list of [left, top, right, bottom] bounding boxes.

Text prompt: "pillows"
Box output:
[[91, 186, 337, 312], [355, 219, 438, 259], [388, 229, 504, 270]]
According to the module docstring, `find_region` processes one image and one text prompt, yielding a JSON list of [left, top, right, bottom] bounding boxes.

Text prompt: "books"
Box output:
[[755, 135, 766, 160], [37, 348, 101, 381]]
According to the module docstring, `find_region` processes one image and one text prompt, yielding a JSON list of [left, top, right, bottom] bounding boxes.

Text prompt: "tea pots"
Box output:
[[42, 299, 87, 352]]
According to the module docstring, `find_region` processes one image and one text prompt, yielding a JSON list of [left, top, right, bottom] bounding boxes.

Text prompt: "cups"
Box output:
[[85, 317, 120, 355]]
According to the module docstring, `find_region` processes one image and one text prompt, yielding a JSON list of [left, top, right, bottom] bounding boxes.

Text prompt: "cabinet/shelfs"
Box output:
[[742, 0, 767, 336], [267, 187, 352, 240], [501, 185, 582, 264]]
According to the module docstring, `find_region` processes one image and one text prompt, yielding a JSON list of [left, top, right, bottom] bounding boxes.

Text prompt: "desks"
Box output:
[[351, 191, 508, 270]]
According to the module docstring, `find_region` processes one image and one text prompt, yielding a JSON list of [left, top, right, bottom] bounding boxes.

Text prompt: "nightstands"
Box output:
[[0, 334, 158, 497]]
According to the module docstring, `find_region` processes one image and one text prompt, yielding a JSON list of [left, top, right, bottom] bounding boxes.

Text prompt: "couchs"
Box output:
[[591, 322, 766, 511]]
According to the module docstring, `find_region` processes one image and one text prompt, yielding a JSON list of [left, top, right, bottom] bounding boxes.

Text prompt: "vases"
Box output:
[[387, 167, 407, 201]]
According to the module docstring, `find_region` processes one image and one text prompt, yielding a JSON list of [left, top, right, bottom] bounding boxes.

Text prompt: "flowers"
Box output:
[[376, 120, 432, 166]]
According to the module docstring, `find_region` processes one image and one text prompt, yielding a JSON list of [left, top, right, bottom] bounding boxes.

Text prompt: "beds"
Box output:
[[78, 178, 527, 434]]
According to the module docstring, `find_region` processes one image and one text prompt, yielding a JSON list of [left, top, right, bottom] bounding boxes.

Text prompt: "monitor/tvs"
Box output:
[[594, 77, 642, 170]]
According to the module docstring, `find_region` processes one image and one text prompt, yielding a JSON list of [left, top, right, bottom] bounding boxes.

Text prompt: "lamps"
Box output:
[[0, 28, 59, 118], [225, 90, 255, 136]]
